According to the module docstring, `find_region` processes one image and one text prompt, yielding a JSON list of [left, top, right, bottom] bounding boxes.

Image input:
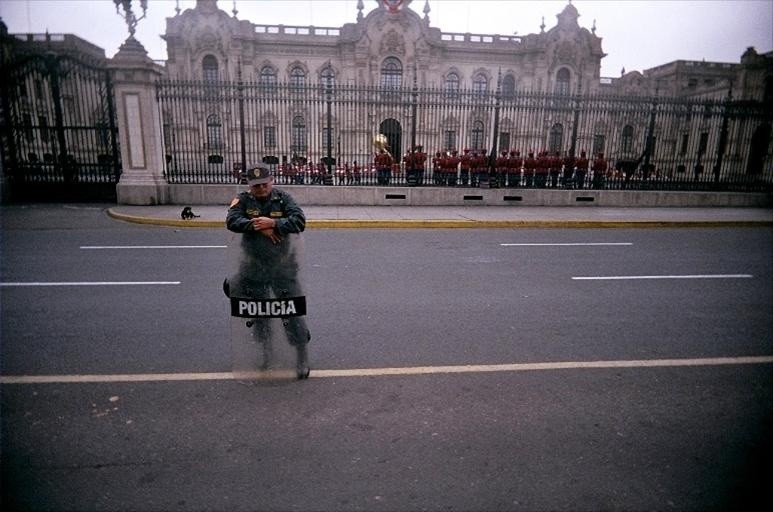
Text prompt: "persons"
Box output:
[[232, 164, 240, 183], [222, 161, 313, 383]]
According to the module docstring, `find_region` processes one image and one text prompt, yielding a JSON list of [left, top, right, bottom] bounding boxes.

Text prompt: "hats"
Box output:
[[405, 144, 587, 154], [246, 162, 273, 188]]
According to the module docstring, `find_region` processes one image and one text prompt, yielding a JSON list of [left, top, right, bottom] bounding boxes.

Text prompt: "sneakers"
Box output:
[[295, 344, 310, 378]]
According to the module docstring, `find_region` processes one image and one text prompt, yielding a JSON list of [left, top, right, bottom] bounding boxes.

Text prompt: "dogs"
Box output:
[[181, 206, 200, 220]]
